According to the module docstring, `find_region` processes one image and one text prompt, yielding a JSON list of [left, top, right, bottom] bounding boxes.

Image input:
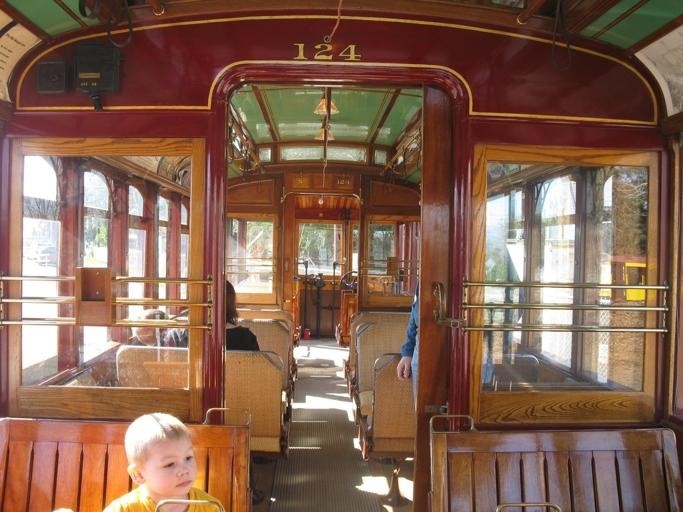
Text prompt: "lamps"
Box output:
[[314, 119, 335, 141], [314, 93, 339, 115]]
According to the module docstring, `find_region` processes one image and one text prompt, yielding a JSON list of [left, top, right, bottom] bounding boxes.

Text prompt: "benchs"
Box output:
[[0, 408, 255, 512], [341, 309, 540, 509], [424, 410, 681, 512], [112, 309, 298, 464]]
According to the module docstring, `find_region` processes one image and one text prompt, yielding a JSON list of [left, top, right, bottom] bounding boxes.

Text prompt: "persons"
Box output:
[[98, 409, 224, 512], [178, 278, 259, 350], [126, 308, 173, 346], [394, 230, 530, 420]]
